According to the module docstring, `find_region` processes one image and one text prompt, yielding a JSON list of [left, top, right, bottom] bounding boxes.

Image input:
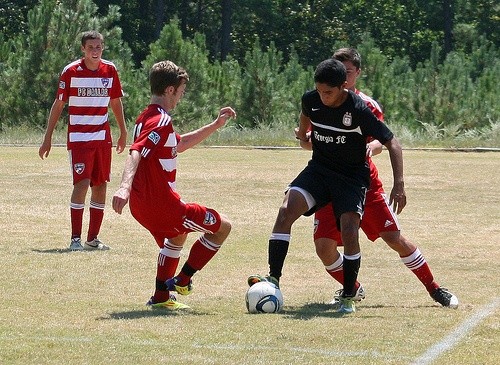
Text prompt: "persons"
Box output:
[[299, 48, 460, 309], [38, 30, 128, 254], [247, 60, 407, 314], [111, 61, 238, 314]]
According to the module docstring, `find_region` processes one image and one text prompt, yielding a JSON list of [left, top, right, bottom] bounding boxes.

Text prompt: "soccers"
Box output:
[[244, 281, 285, 314]]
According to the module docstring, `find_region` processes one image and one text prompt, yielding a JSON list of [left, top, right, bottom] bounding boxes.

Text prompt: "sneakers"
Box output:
[[146, 294, 193, 312], [336, 285, 365, 301], [70, 238, 84, 251], [84, 238, 113, 250], [247, 274, 279, 289], [432, 286, 459, 309], [339, 297, 355, 313], [163, 277, 195, 295]]
